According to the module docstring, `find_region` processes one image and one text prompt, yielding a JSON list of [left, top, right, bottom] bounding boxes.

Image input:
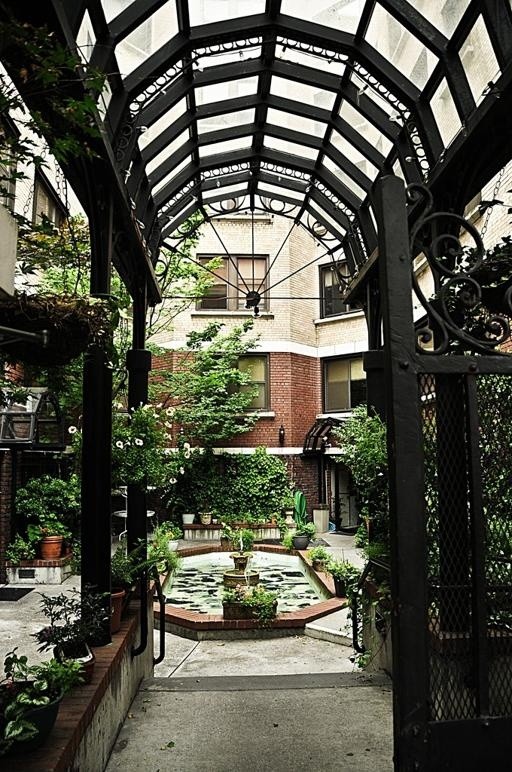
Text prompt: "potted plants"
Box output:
[[291, 522, 361, 597], [223, 526, 257, 572], [221, 591, 278, 622], [1, 579, 126, 757], [182, 490, 215, 524], [13, 474, 79, 560], [156, 521, 184, 552]]
